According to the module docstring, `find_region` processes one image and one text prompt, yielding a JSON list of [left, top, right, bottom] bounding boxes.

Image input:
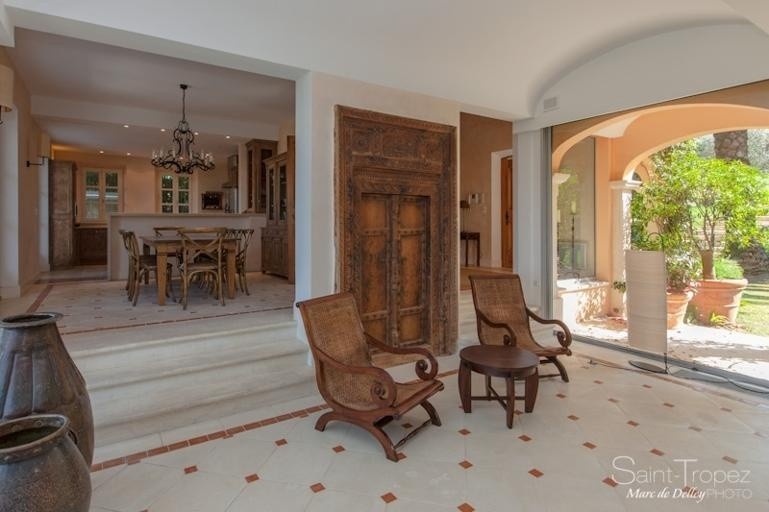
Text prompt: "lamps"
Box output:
[[0, 64, 15, 126], [151, 84, 216, 175], [25, 132, 51, 168], [460, 200, 470, 232]]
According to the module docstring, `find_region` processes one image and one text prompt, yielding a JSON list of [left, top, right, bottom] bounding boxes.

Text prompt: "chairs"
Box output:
[[295, 290, 445, 462], [469, 272, 572, 384], [118, 226, 254, 312]]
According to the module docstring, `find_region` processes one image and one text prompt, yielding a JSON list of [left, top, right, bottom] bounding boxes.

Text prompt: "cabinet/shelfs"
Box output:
[[245, 137, 294, 280], [48, 160, 78, 267]]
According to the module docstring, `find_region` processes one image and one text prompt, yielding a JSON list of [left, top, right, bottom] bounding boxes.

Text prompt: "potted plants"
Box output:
[[628, 148, 768, 331]]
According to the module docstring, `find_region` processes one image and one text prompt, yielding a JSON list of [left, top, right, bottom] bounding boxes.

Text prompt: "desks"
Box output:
[[461, 232, 480, 267]]
